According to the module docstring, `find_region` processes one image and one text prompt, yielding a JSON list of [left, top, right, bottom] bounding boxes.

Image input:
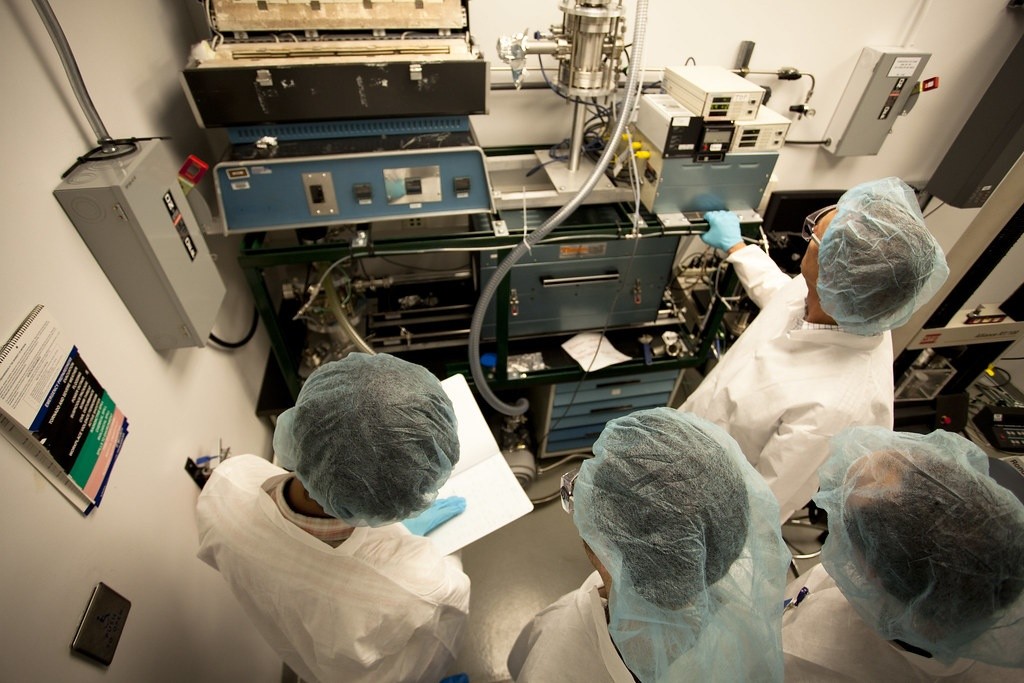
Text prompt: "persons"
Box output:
[[194, 348, 475, 682], [499, 406, 789, 682], [768, 422, 1024, 681], [668, 176, 952, 528]]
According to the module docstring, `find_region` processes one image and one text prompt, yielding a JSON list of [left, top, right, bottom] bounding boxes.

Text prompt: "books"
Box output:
[[1, 304, 132, 516]]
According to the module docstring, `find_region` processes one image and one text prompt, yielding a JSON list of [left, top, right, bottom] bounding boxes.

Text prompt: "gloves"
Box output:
[[701, 210, 744, 254], [399, 495, 465, 536]]
